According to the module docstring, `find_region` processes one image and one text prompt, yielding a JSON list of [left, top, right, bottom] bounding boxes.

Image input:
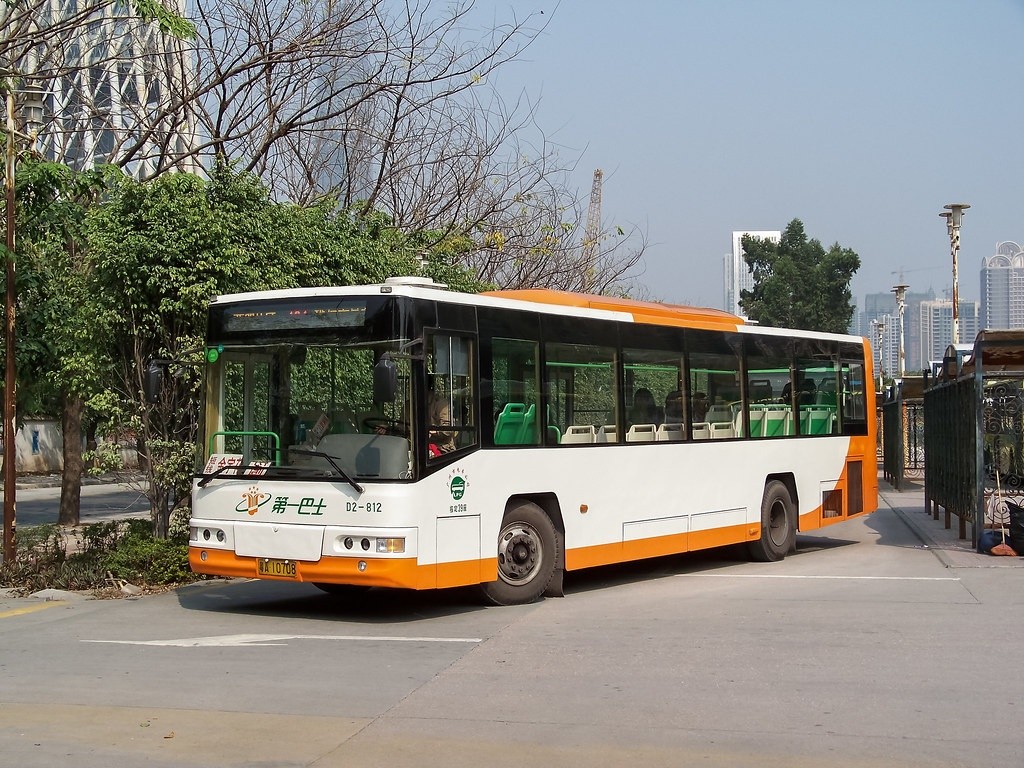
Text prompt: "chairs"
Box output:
[[738, 404, 764, 438], [327, 401, 358, 433], [597, 425, 630, 443], [355, 403, 384, 435], [494, 403, 525, 444], [628, 424, 660, 441], [764, 405, 787, 437], [693, 422, 712, 439], [520, 404, 550, 444], [295, 400, 325, 446], [788, 406, 812, 436], [811, 405, 832, 435], [816, 377, 837, 420], [705, 405, 734, 423], [561, 425, 596, 444], [657, 424, 684, 441], [713, 423, 736, 439], [750, 380, 772, 402]]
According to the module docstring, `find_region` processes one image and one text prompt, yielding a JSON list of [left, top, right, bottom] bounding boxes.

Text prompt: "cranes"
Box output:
[[579, 168, 603, 293]]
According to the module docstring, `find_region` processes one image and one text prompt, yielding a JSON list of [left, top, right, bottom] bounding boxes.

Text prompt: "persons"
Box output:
[[778, 359, 818, 409], [373, 369, 457, 458], [626, 386, 711, 434]]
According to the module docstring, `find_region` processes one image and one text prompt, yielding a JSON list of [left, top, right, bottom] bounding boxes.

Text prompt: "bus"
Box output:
[[982, 379, 1024, 480], [188, 276, 879, 607]]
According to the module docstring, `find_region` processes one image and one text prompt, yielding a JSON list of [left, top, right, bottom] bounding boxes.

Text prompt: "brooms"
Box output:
[[990, 470, 1017, 557]]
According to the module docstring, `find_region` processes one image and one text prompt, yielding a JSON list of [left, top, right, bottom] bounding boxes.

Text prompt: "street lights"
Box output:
[[890, 284, 911, 377], [878, 323, 888, 394], [3, 75, 55, 561], [939, 204, 971, 345]]
